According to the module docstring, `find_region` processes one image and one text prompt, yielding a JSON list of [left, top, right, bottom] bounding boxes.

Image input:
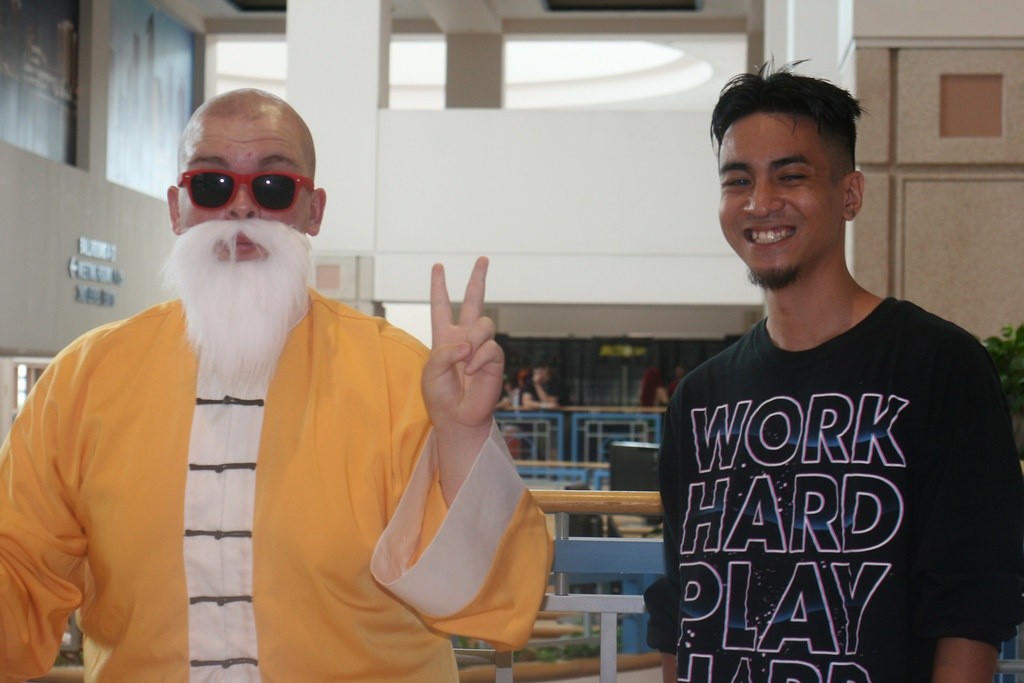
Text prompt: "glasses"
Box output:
[[178, 169, 314, 212]]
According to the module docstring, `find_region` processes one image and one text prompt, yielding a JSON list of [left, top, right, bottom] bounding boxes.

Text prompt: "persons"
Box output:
[[497, 363, 687, 461], [0, 86, 555, 682], [646, 70, 1024, 683]]
